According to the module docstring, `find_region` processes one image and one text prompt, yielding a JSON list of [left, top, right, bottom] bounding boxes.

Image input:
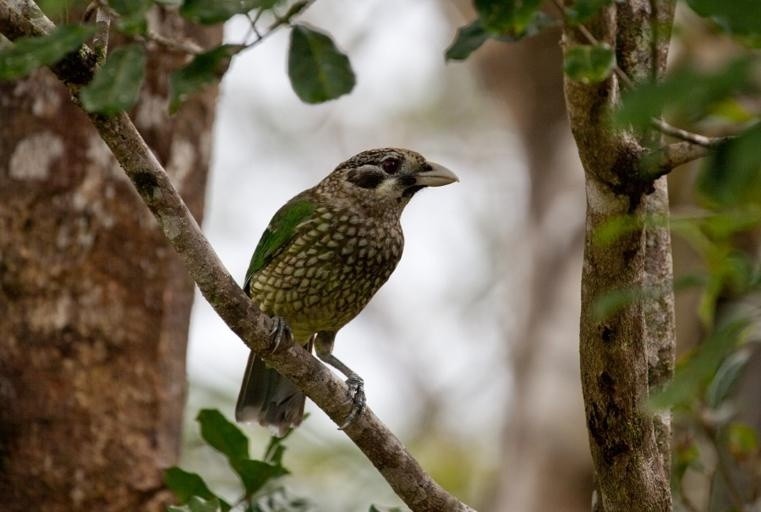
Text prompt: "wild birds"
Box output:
[[234, 147, 460, 436]]
